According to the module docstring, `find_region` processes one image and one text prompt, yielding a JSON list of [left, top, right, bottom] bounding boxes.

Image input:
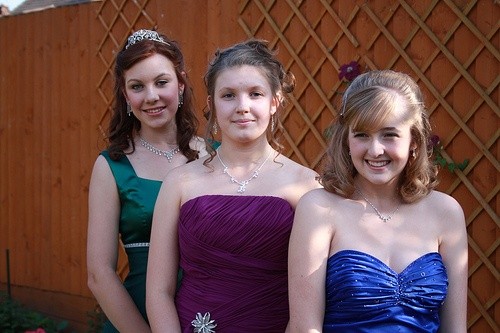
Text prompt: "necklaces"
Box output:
[[353, 184, 405, 223], [138, 132, 181, 163], [214, 146, 273, 193]]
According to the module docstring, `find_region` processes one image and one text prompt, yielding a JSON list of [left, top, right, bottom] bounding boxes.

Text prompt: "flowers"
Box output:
[[338, 60, 362, 84]]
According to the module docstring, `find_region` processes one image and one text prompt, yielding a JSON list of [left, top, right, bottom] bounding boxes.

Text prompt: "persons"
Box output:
[[287, 71, 468, 333], [146, 39, 324, 333], [85, 31, 220, 333]]
